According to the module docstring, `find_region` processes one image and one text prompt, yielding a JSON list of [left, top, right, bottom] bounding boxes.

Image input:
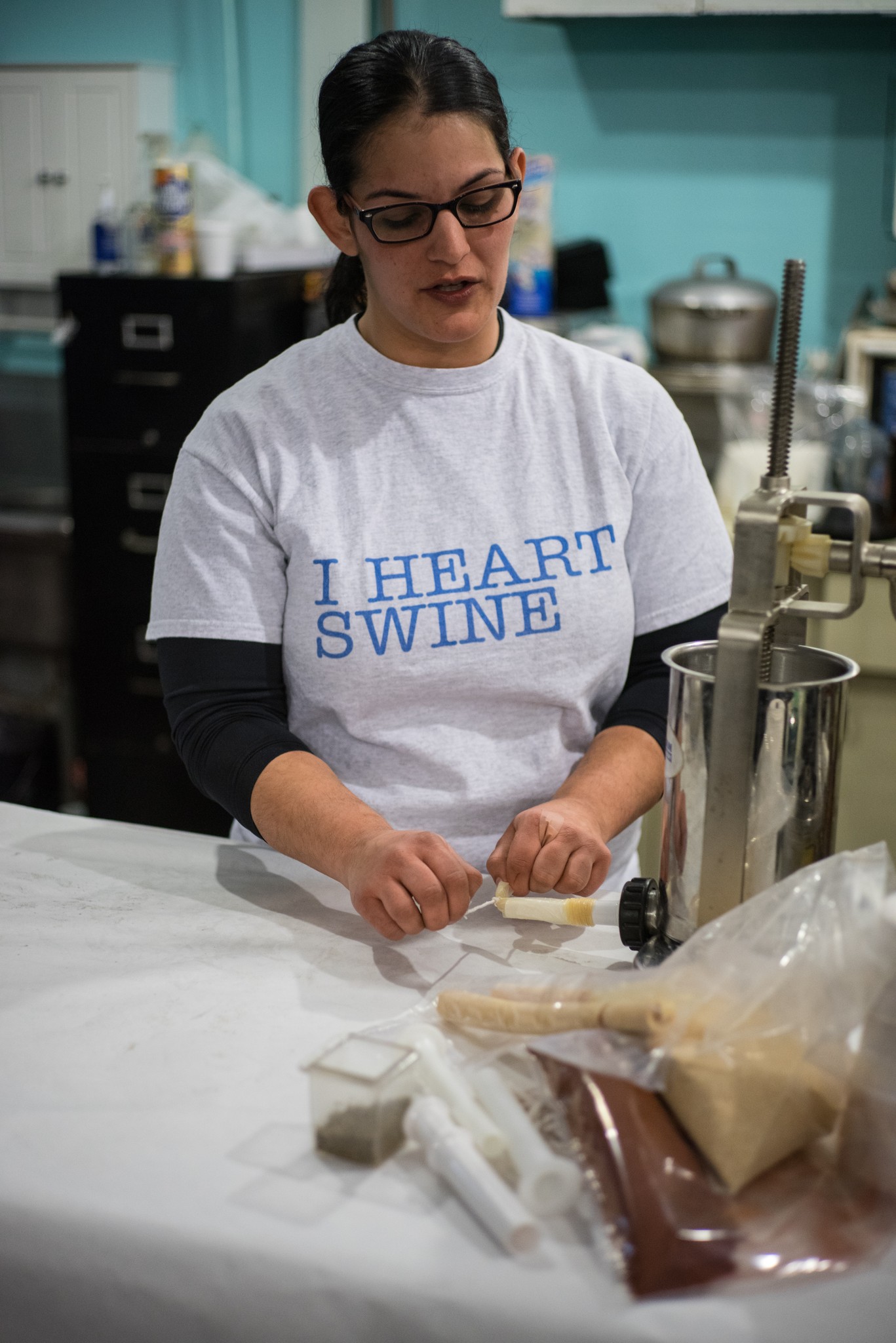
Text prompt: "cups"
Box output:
[[193, 223, 237, 278]]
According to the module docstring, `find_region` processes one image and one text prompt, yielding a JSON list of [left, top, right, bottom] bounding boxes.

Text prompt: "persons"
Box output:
[[145, 28, 735, 941]]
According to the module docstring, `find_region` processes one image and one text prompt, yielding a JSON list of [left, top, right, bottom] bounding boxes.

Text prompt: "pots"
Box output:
[[650, 252, 778, 364]]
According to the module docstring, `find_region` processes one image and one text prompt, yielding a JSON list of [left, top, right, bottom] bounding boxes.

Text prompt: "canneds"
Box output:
[[154, 159, 195, 279]]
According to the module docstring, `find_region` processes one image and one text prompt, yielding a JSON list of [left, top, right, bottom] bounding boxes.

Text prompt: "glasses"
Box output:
[[340, 161, 522, 244]]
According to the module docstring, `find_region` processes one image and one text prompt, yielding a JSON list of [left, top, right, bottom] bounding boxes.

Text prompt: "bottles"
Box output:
[[92, 189, 126, 273], [126, 199, 160, 275]]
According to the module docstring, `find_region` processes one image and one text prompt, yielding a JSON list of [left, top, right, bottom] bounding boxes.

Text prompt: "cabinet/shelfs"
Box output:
[[0, 63, 174, 291], [57, 265, 333, 837]]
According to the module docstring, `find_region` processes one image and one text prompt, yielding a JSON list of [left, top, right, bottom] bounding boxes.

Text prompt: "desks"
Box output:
[[0, 803, 896, 1343]]
